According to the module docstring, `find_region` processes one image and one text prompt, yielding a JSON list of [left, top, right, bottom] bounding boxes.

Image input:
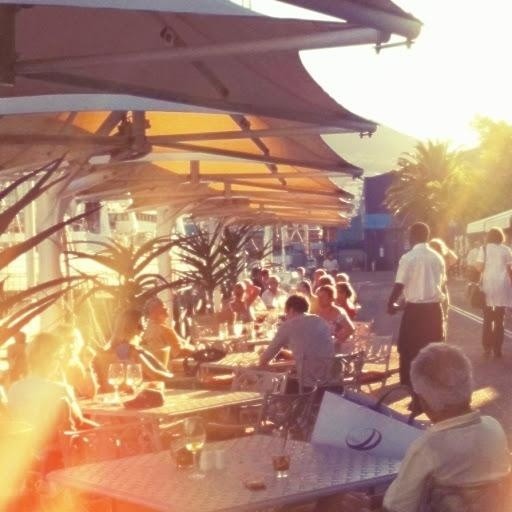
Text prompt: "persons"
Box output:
[[112, 308, 173, 382], [425, 238, 459, 346], [230, 264, 359, 351], [257, 294, 344, 401], [474, 226, 511, 357], [4, 331, 98, 459], [463, 240, 480, 288], [48, 325, 98, 401], [385, 220, 450, 389], [322, 341, 511, 512], [138, 296, 196, 357]]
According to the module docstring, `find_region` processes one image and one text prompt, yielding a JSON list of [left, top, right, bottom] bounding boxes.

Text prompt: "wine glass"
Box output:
[[124, 364, 144, 402], [233, 319, 243, 339], [183, 415, 207, 481], [107, 362, 125, 405]]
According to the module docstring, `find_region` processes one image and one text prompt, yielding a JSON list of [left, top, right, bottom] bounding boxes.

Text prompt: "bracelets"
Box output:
[[84, 367, 92, 372]]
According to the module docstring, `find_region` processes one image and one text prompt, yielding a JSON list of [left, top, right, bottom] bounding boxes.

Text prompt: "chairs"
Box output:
[[7, 303, 511, 511]]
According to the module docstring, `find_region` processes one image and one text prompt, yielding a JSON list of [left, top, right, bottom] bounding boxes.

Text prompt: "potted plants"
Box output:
[[171, 215, 232, 337]]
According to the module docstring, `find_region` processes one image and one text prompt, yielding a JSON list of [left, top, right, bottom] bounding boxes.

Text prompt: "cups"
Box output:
[[218, 321, 228, 341], [270, 429, 291, 480], [245, 321, 255, 339]]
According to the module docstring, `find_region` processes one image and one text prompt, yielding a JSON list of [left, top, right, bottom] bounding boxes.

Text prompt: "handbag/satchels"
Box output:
[[308, 382, 429, 457], [466, 282, 488, 311], [194, 340, 230, 363]]
